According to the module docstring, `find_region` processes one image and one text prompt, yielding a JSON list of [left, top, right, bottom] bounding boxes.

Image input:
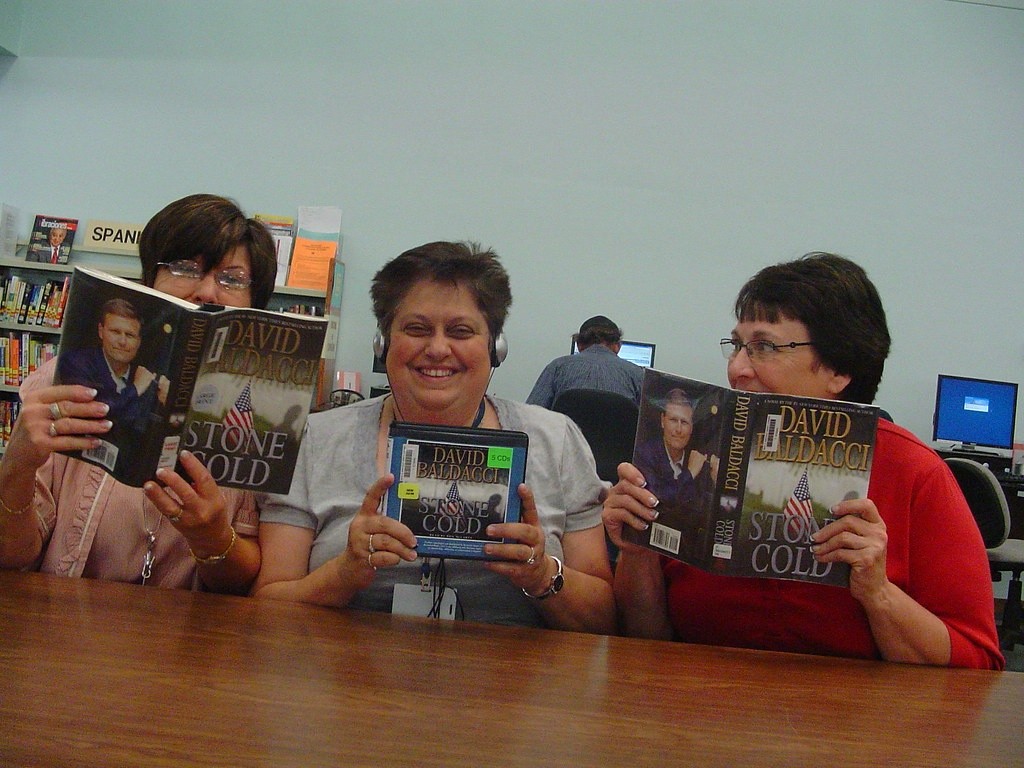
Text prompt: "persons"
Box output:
[[27, 226, 67, 263], [61, 299, 170, 424], [526, 316, 642, 405], [0, 194, 278, 597], [250, 241, 614, 633], [603, 253, 1006, 673], [637, 389, 720, 506]]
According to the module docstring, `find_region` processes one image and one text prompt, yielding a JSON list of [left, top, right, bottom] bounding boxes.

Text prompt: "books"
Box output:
[[381, 421, 529, 562], [622, 367, 879, 590], [1, 276, 70, 447], [52, 269, 328, 497], [279, 304, 324, 318]]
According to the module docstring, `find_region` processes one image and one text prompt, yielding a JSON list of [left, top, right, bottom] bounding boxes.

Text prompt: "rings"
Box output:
[[51, 423, 56, 437], [369, 535, 375, 553], [171, 510, 182, 522], [528, 546, 535, 565], [51, 402, 62, 420], [368, 555, 371, 564]]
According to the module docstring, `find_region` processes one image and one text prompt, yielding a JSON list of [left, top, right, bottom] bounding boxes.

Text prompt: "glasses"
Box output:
[[156, 259, 255, 291], [719, 338, 817, 360]]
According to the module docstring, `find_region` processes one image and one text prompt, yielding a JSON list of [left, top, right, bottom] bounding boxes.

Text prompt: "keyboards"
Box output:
[[993, 472, 1024, 483]]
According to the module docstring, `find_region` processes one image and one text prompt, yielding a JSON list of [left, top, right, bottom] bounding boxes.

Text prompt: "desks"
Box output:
[[0, 563, 1024, 768]]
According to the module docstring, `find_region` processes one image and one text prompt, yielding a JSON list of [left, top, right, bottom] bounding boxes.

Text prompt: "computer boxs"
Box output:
[[933, 446, 1014, 477]]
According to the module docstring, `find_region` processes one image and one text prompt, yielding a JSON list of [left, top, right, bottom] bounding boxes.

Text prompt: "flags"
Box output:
[[223, 383, 253, 443], [784, 471, 813, 532]]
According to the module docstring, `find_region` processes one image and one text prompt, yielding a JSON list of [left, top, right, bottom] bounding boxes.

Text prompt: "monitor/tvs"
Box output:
[[933, 375, 1019, 457], [571, 338, 655, 367]]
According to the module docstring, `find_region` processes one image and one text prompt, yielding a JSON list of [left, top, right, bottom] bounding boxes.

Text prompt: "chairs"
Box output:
[[942, 456, 1024, 651]]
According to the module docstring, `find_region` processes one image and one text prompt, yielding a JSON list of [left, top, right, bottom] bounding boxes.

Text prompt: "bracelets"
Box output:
[[0, 477, 38, 514]]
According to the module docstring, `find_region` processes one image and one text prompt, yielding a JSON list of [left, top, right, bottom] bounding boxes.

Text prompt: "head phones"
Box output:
[[372, 328, 508, 367]]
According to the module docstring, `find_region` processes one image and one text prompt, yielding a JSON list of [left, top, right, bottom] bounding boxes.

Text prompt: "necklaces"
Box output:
[[140, 492, 163, 587]]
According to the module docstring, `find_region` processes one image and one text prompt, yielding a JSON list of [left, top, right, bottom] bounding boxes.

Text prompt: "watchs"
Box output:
[[522, 556, 564, 599], [189, 527, 237, 564]]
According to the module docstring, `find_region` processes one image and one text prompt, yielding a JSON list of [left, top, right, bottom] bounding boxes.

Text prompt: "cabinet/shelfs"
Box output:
[[0, 257, 75, 449]]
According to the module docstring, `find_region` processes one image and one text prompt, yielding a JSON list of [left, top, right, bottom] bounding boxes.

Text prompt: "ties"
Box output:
[[51, 248, 58, 264]]
